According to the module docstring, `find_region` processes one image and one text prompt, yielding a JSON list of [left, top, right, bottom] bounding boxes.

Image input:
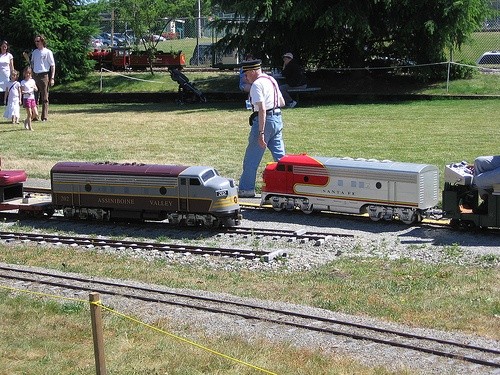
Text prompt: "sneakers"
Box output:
[[237, 189, 256, 198]]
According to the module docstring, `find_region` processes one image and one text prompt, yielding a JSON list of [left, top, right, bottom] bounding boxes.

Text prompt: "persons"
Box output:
[[237, 58, 286, 198], [466, 155, 500, 213], [0, 33, 56, 132], [239, 53, 308, 107]]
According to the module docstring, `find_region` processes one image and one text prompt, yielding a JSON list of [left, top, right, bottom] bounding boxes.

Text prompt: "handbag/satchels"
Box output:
[[249, 111, 258, 126]]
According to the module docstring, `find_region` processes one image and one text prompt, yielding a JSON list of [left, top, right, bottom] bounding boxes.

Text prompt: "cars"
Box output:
[[477, 51, 500, 72], [87, 30, 166, 51]]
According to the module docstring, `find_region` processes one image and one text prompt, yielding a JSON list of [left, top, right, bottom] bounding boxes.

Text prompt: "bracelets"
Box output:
[[50, 78, 54, 80], [258, 130, 264, 135]]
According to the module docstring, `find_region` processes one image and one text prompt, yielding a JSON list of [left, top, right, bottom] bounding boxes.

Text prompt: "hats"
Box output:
[[242, 60, 262, 73], [283, 53, 293, 59]]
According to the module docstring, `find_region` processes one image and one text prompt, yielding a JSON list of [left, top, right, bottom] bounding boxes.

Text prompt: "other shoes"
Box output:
[[289, 101, 297, 108]]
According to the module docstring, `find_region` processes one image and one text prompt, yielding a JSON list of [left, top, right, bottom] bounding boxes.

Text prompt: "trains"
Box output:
[[189, 44, 277, 72], [261, 154, 500, 231], [90, 46, 186, 72], [0, 160, 243, 229]]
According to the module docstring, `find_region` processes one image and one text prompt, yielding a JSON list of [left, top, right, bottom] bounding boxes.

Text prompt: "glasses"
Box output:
[[34, 41, 40, 42]]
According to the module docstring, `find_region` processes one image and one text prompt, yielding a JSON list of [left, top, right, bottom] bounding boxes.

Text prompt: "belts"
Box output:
[[267, 112, 282, 115]]
